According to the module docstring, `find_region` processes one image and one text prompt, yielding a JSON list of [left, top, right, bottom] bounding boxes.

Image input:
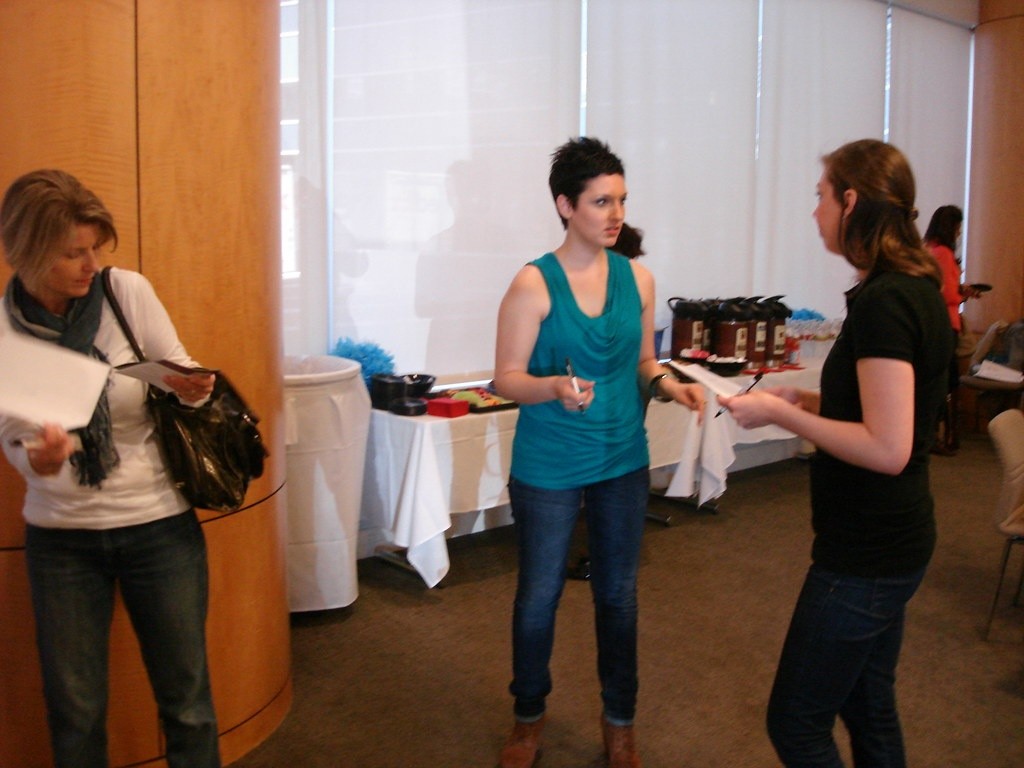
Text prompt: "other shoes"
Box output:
[[931, 438, 951, 456], [499, 715, 544, 768], [601, 711, 643, 768]]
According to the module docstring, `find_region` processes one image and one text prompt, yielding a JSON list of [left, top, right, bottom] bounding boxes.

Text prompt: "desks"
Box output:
[[357, 364, 705, 588], [650, 334, 838, 514]]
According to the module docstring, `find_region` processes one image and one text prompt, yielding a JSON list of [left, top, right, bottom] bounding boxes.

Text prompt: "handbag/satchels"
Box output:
[[101, 266, 269, 514]]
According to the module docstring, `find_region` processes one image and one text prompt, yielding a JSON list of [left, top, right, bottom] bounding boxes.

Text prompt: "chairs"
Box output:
[[984, 410, 1023, 646]]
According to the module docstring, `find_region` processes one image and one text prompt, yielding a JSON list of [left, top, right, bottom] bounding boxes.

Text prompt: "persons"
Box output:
[[718, 140, 957, 768], [494, 137, 705, 768], [1, 169, 221, 767], [922, 206, 981, 454]]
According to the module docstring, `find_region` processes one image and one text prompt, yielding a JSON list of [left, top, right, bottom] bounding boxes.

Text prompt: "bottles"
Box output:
[[786, 319, 843, 366]]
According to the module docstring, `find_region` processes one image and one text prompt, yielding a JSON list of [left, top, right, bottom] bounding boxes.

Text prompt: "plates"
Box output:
[[391, 398, 427, 416], [967, 282, 992, 291]]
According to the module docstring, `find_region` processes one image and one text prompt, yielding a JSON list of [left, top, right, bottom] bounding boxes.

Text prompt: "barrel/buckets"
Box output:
[[282, 354, 373, 613]]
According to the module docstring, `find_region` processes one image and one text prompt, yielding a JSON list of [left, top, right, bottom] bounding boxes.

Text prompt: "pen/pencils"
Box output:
[[713, 371, 764, 421], [564, 358, 586, 417]]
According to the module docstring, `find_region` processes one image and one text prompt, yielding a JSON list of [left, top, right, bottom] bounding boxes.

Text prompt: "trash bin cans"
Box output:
[[281, 353, 371, 614]]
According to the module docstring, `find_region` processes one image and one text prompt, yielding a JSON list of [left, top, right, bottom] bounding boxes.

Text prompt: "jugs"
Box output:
[[668, 294, 792, 370]]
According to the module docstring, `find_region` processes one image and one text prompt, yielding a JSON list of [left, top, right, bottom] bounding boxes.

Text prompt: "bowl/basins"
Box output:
[[400, 374, 436, 397], [680, 356, 706, 367], [706, 358, 748, 377]]
